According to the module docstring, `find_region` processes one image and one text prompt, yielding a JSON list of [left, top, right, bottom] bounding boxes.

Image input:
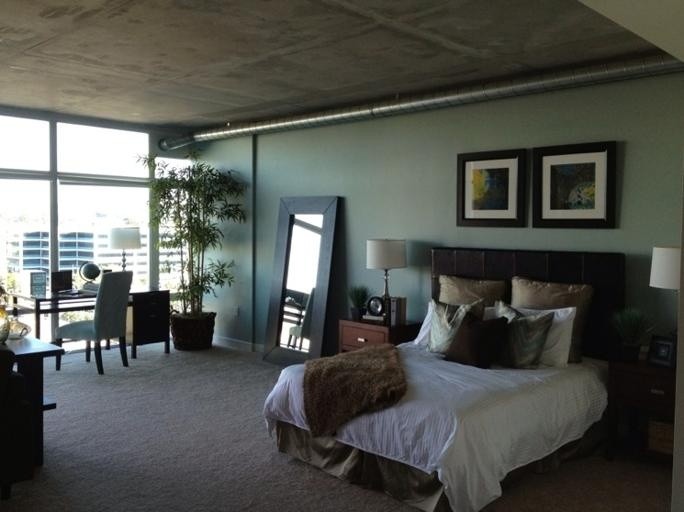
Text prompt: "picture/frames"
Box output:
[[645, 333, 676, 370], [455, 140, 619, 230]]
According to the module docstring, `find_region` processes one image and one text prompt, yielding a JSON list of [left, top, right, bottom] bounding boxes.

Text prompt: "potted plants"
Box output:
[[135, 146, 249, 353], [611, 307, 661, 361], [347, 284, 372, 321]]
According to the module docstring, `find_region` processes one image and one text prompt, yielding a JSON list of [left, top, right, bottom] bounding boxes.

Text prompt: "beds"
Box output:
[[267, 247, 624, 512]]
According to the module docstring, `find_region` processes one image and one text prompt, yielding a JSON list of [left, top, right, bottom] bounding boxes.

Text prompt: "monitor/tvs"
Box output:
[[51, 270, 72, 294]]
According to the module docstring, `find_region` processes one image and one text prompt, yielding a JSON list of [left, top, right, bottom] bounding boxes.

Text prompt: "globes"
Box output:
[[79, 261, 100, 291]]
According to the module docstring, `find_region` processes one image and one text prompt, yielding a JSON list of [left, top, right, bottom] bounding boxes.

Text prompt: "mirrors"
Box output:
[[262, 196, 340, 367]]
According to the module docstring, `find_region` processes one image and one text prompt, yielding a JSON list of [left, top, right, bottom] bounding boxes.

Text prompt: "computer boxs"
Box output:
[[29, 270, 46, 296]]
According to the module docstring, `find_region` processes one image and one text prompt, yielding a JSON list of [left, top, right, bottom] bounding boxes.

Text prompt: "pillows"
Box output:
[[426, 275, 596, 369]]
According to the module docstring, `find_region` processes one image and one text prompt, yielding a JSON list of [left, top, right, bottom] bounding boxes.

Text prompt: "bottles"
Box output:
[[7, 296, 31, 341]]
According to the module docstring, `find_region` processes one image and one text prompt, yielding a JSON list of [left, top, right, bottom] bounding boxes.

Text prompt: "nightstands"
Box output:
[[607, 373, 676, 452], [337, 318, 407, 354]]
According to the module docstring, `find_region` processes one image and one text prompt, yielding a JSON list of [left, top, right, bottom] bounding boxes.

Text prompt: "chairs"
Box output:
[[55, 271, 136, 375], [287, 288, 315, 350]]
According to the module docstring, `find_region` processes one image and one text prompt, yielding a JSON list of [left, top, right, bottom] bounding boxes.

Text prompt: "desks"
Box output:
[[0, 332, 66, 500], [285, 289, 309, 347], [8, 289, 171, 360]]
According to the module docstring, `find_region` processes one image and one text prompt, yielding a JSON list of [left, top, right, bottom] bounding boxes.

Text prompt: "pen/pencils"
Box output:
[[71, 293, 83, 295]]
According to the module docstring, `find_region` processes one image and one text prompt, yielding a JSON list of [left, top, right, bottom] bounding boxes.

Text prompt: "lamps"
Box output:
[[110, 227, 142, 272], [649, 246, 681, 331], [365, 239, 407, 297]]
